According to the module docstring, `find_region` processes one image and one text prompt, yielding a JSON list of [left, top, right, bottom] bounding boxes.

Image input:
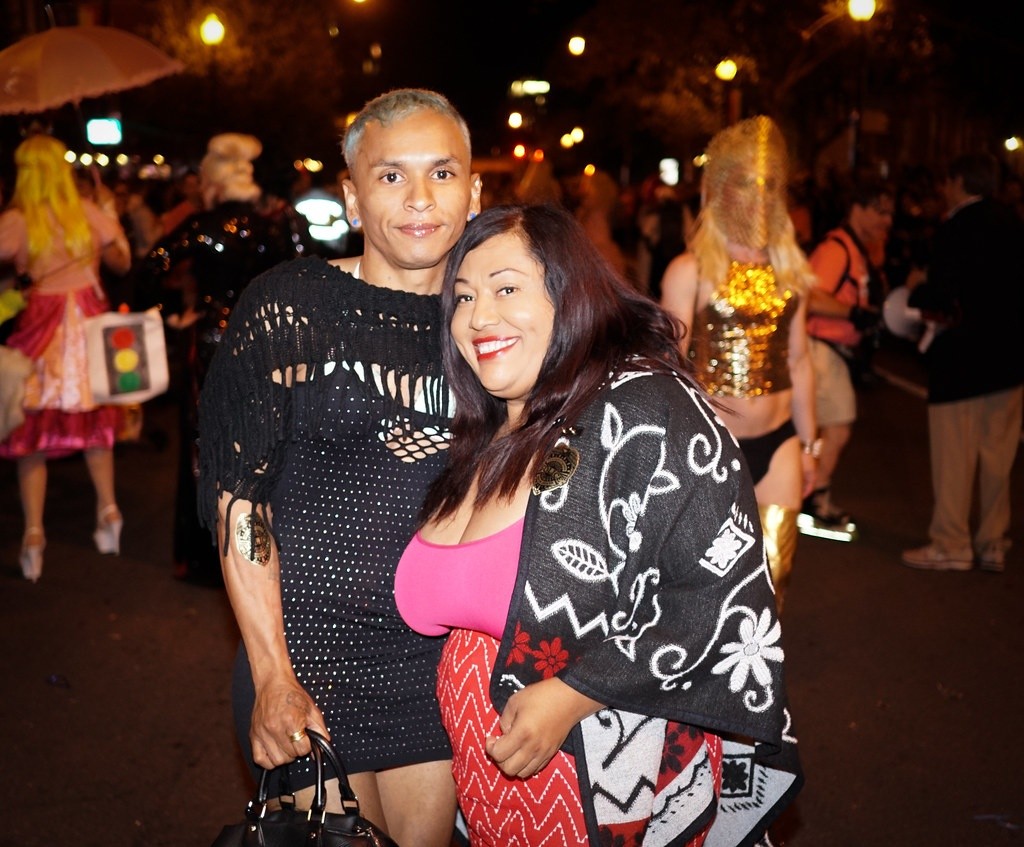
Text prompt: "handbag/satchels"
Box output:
[[210, 727, 400, 847]]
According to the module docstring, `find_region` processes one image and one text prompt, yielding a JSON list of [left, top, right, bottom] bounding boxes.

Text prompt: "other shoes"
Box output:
[[900, 547, 972, 570], [972, 538, 1006, 572], [796, 487, 858, 542]]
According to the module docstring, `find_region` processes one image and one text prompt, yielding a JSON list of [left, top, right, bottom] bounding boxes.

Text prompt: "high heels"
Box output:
[[19, 526, 47, 581], [92, 504, 122, 555]]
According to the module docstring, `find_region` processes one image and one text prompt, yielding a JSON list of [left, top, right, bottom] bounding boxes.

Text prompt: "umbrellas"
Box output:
[[1, 3, 189, 116]]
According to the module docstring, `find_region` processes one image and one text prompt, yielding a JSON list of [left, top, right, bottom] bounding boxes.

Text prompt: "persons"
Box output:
[[2, 129, 1022, 586], [193, 86, 802, 840]]
